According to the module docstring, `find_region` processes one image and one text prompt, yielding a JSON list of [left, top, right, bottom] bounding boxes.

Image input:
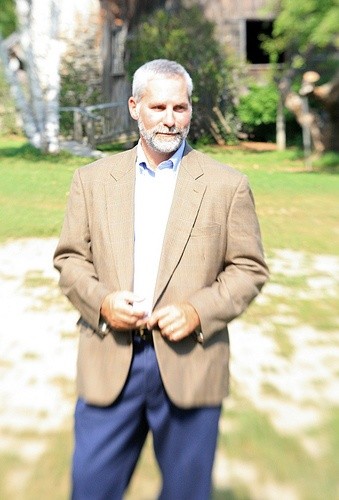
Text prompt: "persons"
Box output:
[[52, 59, 270, 500]]
